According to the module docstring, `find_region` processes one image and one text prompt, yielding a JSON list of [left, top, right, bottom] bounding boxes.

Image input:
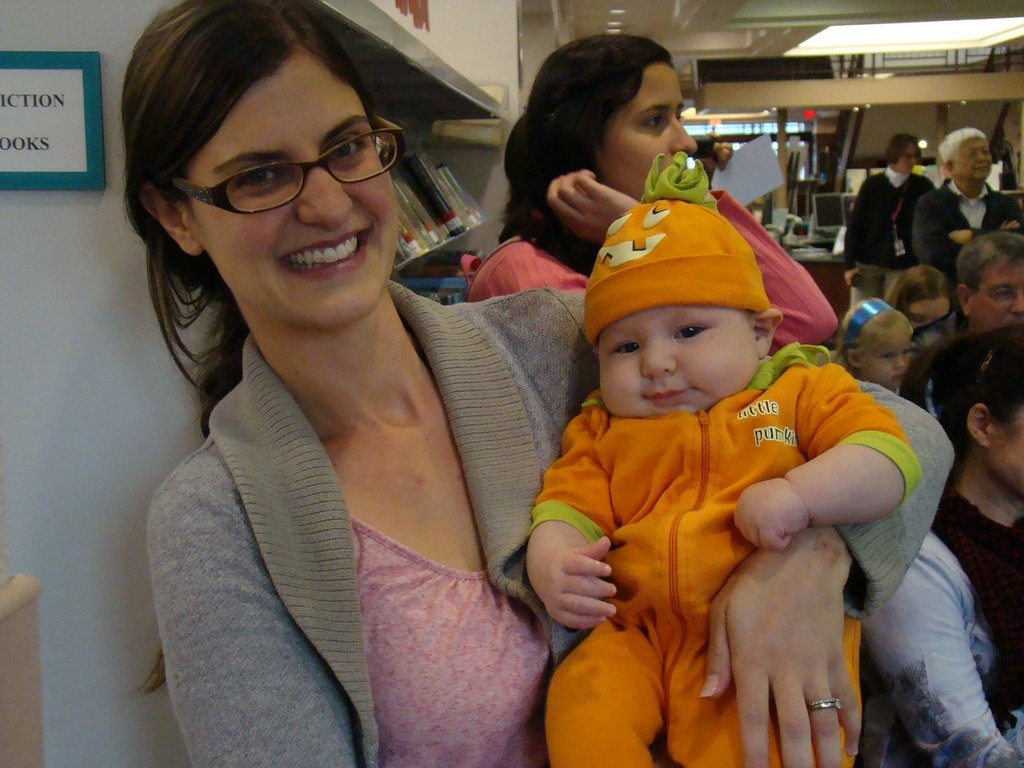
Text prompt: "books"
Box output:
[[387, 152, 486, 261]]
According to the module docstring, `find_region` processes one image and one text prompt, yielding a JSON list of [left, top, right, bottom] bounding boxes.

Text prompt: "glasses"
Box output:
[[171, 113, 406, 214], [970, 287, 1024, 303]]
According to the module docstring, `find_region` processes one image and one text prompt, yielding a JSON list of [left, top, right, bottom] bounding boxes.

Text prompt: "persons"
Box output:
[[121, 0, 954, 768], [913, 128, 1024, 283], [902, 231, 1024, 415], [933, 324, 1024, 716], [844, 133, 935, 299], [888, 267, 948, 328], [471, 35, 839, 356], [834, 298, 913, 394], [524, 151, 923, 767]]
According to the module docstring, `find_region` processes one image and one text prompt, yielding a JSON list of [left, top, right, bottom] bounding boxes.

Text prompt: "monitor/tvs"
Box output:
[[813, 192, 845, 234]]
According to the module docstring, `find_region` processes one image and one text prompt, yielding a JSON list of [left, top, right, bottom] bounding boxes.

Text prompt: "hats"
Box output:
[[582, 152, 770, 351]]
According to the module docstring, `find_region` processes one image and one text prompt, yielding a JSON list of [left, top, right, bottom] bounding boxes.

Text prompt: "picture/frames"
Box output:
[[0, 51, 107, 190]]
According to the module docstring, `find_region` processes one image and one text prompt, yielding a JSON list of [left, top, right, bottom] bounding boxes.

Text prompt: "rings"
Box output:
[[808, 698, 841, 711]]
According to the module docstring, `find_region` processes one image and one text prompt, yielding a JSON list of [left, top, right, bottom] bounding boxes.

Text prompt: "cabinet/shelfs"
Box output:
[[0, 1, 518, 768]]
